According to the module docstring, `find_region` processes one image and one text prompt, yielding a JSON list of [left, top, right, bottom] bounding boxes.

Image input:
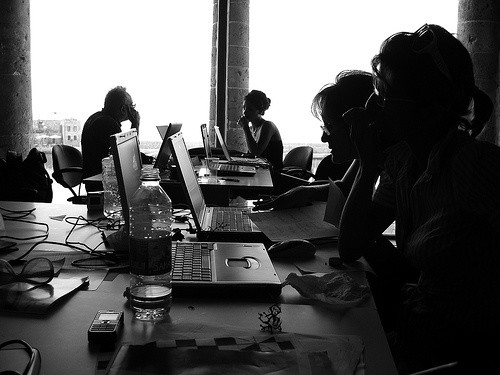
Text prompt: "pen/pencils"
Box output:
[[222, 177, 241, 182]]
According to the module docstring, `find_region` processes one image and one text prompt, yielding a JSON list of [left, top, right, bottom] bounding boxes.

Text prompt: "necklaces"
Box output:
[[251, 118, 265, 136]]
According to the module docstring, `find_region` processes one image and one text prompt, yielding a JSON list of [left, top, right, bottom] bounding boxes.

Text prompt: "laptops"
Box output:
[[109, 122, 282, 298]]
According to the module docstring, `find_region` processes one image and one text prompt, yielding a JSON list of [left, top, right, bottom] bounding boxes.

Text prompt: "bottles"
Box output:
[[126, 165, 174, 323], [101, 148, 123, 220]]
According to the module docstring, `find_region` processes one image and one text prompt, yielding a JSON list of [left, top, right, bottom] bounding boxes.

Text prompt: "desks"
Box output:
[[0, 201, 399, 374], [81, 156, 275, 202]]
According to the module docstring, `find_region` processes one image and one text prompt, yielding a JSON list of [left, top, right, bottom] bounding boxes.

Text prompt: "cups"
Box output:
[[205, 157, 219, 184]]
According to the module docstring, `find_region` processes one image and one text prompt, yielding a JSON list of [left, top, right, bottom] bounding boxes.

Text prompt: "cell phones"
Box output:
[[87, 309, 124, 342]]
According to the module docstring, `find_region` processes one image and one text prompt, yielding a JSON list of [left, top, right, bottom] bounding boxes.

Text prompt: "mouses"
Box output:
[[268, 238, 316, 258]]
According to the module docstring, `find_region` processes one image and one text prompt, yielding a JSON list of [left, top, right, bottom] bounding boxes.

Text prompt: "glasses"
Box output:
[[123, 103, 136, 108], [320, 108, 356, 136]]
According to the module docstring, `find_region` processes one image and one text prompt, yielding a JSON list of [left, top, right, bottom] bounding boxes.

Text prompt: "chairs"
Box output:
[[52, 144, 89, 204], [281, 146, 313, 185]]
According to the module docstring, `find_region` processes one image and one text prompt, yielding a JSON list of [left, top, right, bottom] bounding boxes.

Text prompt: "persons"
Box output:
[[81, 85, 156, 196], [337, 22, 500, 375], [236, 89, 284, 198], [252, 66, 393, 214]]
